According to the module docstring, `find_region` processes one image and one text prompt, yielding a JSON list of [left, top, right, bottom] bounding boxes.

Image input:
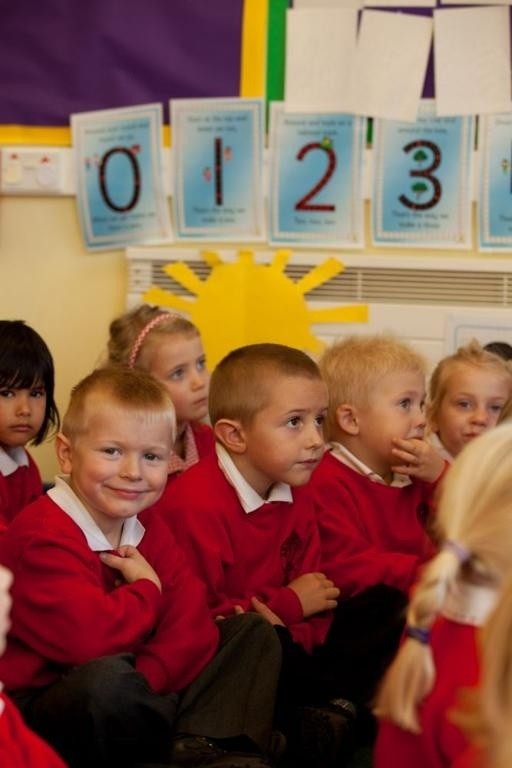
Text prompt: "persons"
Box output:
[[0, 563, 68, 768], [301, 334, 452, 596], [0, 365, 285, 768], [421, 337, 512, 467], [105, 302, 216, 480], [371, 418, 512, 767], [149, 343, 410, 767], [0, 319, 62, 523]]
